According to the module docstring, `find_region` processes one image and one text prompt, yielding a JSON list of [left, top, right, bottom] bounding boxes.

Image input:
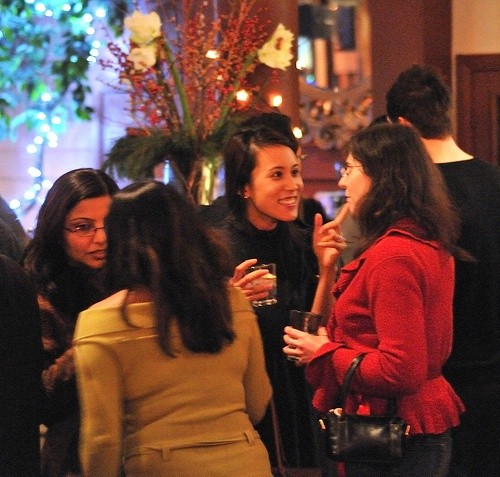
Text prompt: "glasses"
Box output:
[[63, 223, 104, 237], [340, 162, 363, 175], [294, 151, 307, 160]]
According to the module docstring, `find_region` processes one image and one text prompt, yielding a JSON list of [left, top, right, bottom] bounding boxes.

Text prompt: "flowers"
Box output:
[[99, 0, 295, 180]]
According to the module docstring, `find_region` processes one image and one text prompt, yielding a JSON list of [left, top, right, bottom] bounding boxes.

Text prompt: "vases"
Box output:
[[171, 157, 221, 206]]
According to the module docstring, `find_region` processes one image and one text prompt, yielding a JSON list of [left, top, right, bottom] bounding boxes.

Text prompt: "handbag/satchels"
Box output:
[[319, 352, 411, 463]]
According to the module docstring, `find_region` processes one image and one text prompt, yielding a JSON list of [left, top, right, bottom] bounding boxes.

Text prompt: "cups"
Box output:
[[287, 310, 324, 363], [242, 264, 278, 306]]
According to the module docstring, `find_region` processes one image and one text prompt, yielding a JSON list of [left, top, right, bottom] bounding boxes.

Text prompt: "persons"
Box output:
[[368, 63, 500, 477], [0, 168, 121, 477], [197, 112, 363, 477], [72, 180, 273, 477], [283, 125, 466, 477]]
